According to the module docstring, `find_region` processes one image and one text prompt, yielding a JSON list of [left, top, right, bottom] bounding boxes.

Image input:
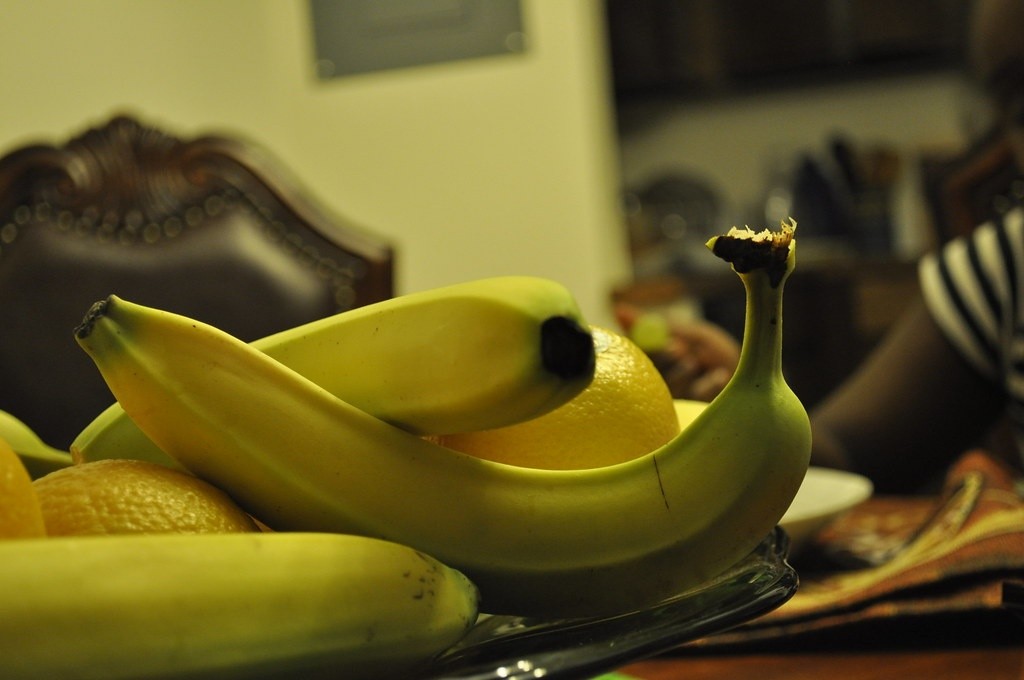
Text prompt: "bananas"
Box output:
[[0, 215, 814, 680]]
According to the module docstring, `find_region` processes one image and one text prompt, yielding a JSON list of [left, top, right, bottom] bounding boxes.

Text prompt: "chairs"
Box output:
[[1, 112, 401, 458]]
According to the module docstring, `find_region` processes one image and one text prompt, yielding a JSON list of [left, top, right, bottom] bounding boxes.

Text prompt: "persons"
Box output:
[[666, 66, 1023, 492]]
